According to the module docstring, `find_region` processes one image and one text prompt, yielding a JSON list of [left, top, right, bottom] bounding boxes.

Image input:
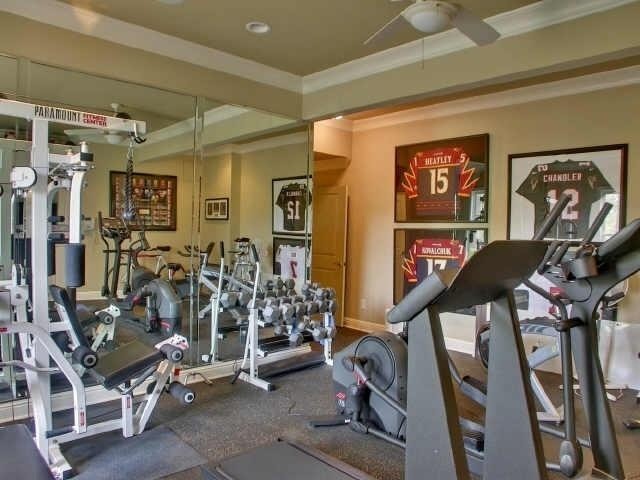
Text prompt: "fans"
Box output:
[[63, 103, 135, 135], [363, 0, 500, 50]]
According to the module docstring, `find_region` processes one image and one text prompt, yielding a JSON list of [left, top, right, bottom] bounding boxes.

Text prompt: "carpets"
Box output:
[[0, 328, 208, 480]]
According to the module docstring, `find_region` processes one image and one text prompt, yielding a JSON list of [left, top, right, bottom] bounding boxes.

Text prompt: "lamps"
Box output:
[[103, 131, 126, 144], [398, 0, 459, 34]]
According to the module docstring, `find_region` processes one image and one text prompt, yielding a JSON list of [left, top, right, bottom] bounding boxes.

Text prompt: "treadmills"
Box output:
[[197, 241, 548, 480]]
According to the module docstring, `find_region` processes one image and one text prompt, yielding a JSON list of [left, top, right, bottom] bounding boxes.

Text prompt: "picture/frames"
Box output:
[[271, 174, 311, 293], [110, 170, 178, 231], [204, 198, 228, 220], [392, 132, 489, 224], [506, 143, 628, 319], [393, 228, 488, 315]]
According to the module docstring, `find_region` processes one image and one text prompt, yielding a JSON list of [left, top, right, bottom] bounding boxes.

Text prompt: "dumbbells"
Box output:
[[236, 314, 251, 327], [211, 279, 296, 307], [247, 286, 336, 347]]
